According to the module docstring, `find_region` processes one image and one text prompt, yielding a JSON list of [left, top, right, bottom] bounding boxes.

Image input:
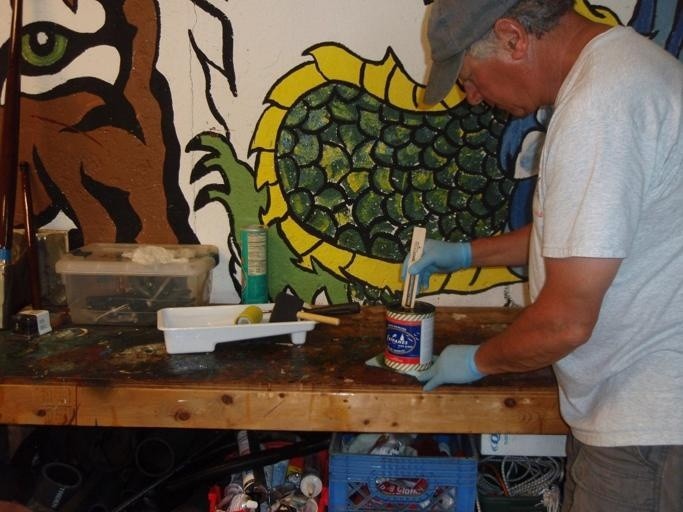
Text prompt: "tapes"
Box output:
[[235, 306, 264, 324]]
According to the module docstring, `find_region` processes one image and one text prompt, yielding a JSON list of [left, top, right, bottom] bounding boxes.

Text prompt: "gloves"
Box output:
[[400, 238, 472, 296], [415, 344, 489, 393]]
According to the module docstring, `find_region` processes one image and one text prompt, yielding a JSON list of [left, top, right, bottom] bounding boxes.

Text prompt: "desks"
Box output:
[[0, 305, 569, 512]]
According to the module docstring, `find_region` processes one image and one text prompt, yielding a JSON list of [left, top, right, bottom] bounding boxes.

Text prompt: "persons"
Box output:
[[401, 0, 683, 512]]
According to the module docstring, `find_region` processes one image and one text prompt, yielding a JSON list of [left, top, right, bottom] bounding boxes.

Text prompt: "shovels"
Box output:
[[269, 291, 339, 326]]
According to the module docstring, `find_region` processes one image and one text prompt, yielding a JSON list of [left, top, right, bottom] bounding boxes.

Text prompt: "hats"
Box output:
[[423, 0, 517, 106]]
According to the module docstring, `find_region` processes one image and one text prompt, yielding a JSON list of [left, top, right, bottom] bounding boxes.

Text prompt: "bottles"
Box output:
[[215, 429, 322, 511], [240, 222, 268, 304], [0, 247, 15, 329]]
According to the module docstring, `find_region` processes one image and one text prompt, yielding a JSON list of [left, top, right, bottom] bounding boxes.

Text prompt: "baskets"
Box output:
[[327, 430, 479, 512]]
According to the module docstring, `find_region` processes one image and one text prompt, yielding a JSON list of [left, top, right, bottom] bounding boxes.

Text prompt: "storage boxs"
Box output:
[[207, 439, 328, 512], [476, 474, 555, 512], [56, 240, 217, 327]]
